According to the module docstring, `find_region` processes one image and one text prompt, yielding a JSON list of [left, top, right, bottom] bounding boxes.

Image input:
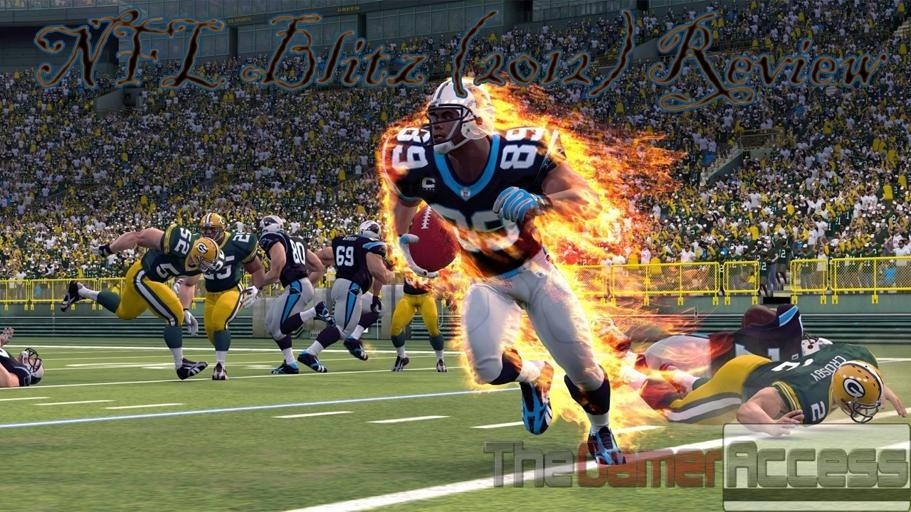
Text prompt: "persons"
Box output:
[[296, 221, 396, 374], [381, 76, 626, 466], [610, 342, 907, 439], [251, 216, 325, 376], [178, 212, 265, 381], [61, 224, 220, 380], [0, 0, 911, 305], [387, 239, 457, 374], [0, 324, 45, 388], [599, 303, 834, 397]]
